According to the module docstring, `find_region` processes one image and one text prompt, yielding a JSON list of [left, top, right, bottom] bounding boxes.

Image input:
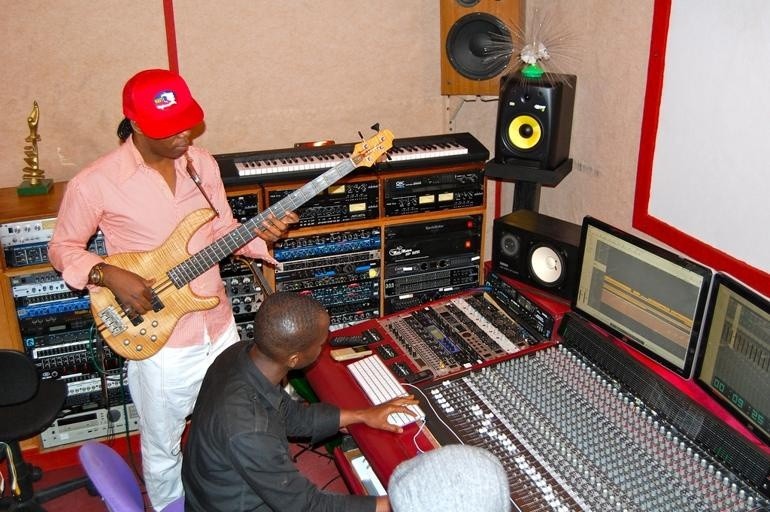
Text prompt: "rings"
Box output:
[[124, 308, 132, 315]]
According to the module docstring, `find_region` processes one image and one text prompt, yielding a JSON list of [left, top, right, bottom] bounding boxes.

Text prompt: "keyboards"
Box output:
[[346, 354, 425, 427]]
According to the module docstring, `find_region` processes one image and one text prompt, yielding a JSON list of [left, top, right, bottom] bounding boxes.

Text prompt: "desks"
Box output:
[[302, 260, 770, 512]]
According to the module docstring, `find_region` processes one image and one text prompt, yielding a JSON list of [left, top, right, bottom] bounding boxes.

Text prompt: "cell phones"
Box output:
[[405, 369, 434, 384]]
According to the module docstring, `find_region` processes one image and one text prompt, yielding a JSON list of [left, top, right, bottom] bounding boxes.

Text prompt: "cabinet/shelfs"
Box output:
[[258, 161, 488, 319], [0, 180, 269, 453]]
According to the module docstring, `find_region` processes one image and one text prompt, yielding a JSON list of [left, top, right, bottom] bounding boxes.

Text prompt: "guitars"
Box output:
[[86, 128, 395, 357]]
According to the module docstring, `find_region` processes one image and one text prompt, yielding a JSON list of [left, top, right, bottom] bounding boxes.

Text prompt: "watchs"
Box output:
[[90, 262, 107, 286]]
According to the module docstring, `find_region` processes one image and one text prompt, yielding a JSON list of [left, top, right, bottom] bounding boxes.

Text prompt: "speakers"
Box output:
[[495, 73, 577, 171], [491, 209, 582, 302], [440, 1, 527, 96]]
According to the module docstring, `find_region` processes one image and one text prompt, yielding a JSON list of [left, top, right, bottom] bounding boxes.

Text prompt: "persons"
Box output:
[[46, 68, 298, 512], [182, 288, 419, 512]]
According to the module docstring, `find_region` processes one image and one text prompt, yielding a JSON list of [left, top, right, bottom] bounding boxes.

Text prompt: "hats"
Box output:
[[122, 70, 205, 139], [388, 445, 510, 512]]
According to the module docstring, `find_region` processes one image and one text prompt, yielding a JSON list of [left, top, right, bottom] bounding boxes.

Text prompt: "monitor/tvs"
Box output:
[[571, 215, 717, 382], [693, 272, 770, 448]]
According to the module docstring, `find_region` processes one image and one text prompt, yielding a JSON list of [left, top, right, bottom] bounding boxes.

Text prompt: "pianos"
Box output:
[[211, 131, 490, 189]]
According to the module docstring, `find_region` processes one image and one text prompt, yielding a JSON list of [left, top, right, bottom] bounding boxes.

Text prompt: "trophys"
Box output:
[[17, 100, 53, 197]]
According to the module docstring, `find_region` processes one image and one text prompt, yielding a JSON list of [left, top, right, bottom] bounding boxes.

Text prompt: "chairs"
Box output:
[[79, 441, 185, 512], [0, 348, 99, 512]]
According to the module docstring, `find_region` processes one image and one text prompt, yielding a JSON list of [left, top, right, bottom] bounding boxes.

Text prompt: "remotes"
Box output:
[[331, 346, 372, 362], [328, 336, 370, 346]]
